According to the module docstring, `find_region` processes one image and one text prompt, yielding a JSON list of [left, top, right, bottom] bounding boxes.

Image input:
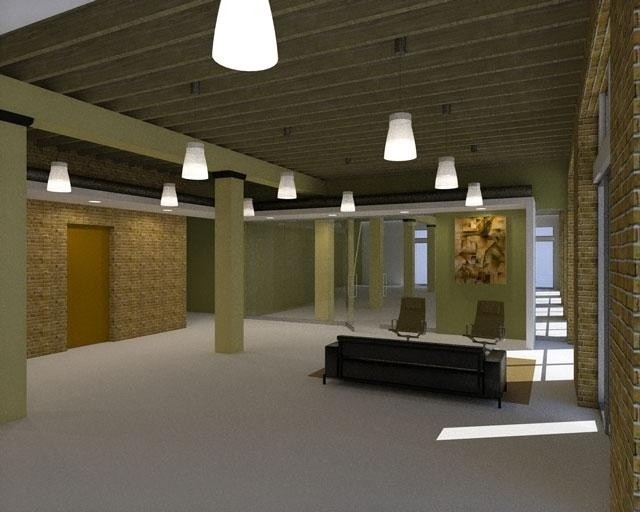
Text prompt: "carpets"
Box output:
[[307, 357, 536, 405]]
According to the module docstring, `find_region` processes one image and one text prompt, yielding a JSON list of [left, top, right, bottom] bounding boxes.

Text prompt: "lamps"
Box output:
[[382, 36, 484, 208], [45, 162, 72, 193], [339, 157, 355, 213], [211, 0, 278, 73], [157, 81, 297, 217]]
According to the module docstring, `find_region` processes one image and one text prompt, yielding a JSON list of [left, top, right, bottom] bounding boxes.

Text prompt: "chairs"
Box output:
[[463, 300, 505, 347], [388, 297, 427, 340]]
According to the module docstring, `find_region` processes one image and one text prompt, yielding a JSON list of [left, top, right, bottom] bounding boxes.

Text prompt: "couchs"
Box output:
[[322, 334, 507, 408]]
